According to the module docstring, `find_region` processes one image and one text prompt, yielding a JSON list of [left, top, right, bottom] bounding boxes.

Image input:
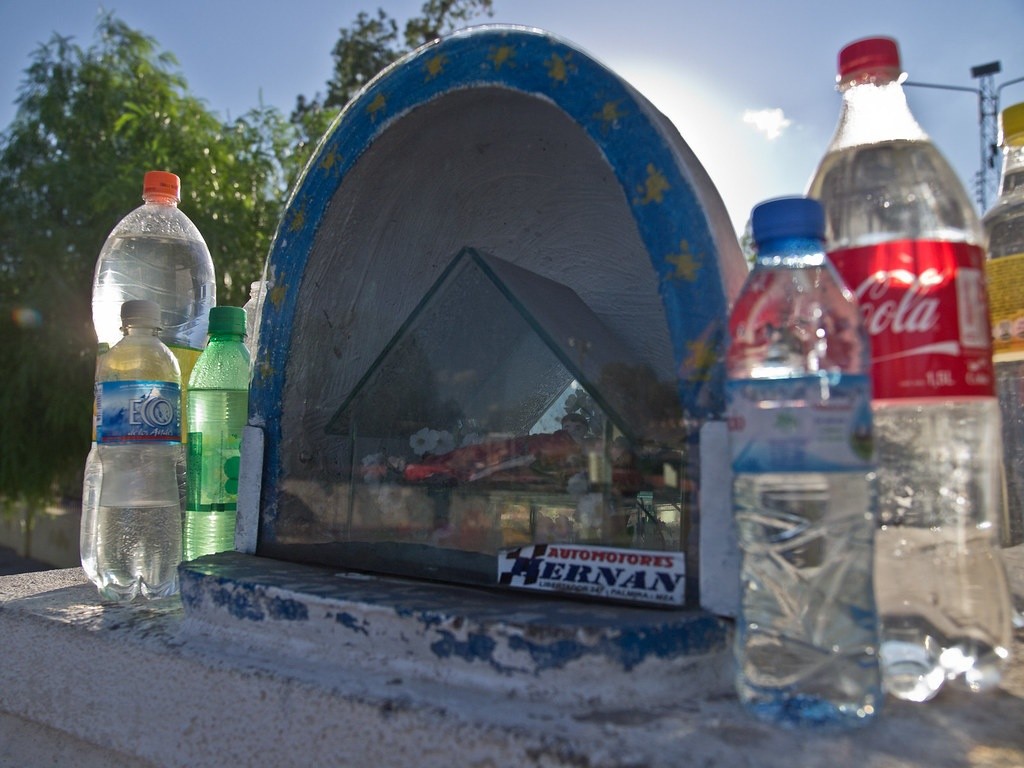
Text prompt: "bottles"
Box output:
[[982, 103, 1024, 696], [241, 282, 267, 354], [97, 300, 182, 601], [809, 39, 1015, 703], [80, 171, 216, 586], [182, 306, 250, 561], [725, 196, 879, 730]]
